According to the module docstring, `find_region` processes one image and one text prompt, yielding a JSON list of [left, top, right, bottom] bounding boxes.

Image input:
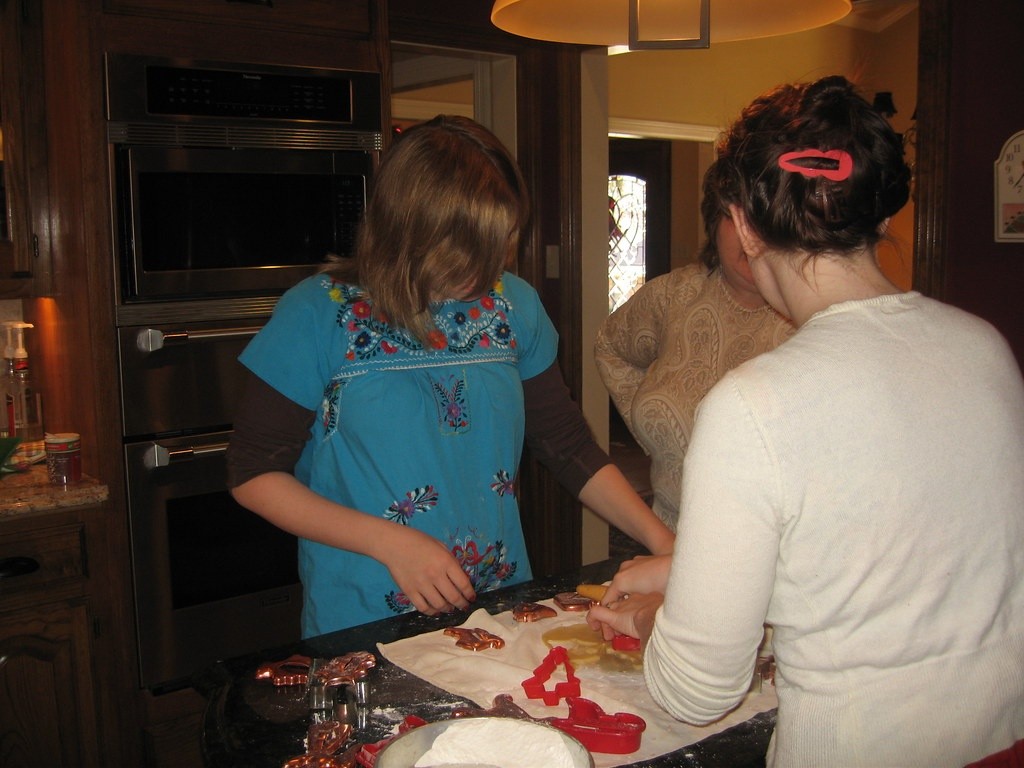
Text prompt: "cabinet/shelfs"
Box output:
[[0, 458, 134, 768]]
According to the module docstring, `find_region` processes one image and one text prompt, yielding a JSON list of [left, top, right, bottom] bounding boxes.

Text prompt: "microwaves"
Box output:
[[103, 51, 384, 303]]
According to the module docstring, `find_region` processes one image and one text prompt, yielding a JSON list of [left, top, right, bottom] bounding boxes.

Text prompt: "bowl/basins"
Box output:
[[374, 717, 595, 768]]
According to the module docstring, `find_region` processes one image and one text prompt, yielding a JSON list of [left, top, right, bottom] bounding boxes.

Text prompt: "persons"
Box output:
[[223, 114, 676, 637], [586, 74, 1024, 768]]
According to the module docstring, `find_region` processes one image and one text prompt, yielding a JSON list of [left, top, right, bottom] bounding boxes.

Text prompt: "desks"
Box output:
[[190, 552, 780, 768]]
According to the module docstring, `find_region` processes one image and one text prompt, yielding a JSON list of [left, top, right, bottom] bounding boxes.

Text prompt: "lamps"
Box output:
[[489, 0, 854, 52]]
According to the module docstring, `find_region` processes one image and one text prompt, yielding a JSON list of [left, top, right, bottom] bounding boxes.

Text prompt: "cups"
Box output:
[[44, 433, 81, 486]]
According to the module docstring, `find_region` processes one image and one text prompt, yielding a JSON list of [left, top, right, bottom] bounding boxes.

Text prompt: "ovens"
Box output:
[[117, 317, 306, 687]]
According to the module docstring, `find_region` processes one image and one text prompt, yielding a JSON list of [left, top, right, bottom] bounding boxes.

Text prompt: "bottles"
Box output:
[[0, 358, 42, 444]]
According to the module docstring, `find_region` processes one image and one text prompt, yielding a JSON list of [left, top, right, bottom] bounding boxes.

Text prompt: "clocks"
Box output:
[[992, 130, 1024, 243]]
